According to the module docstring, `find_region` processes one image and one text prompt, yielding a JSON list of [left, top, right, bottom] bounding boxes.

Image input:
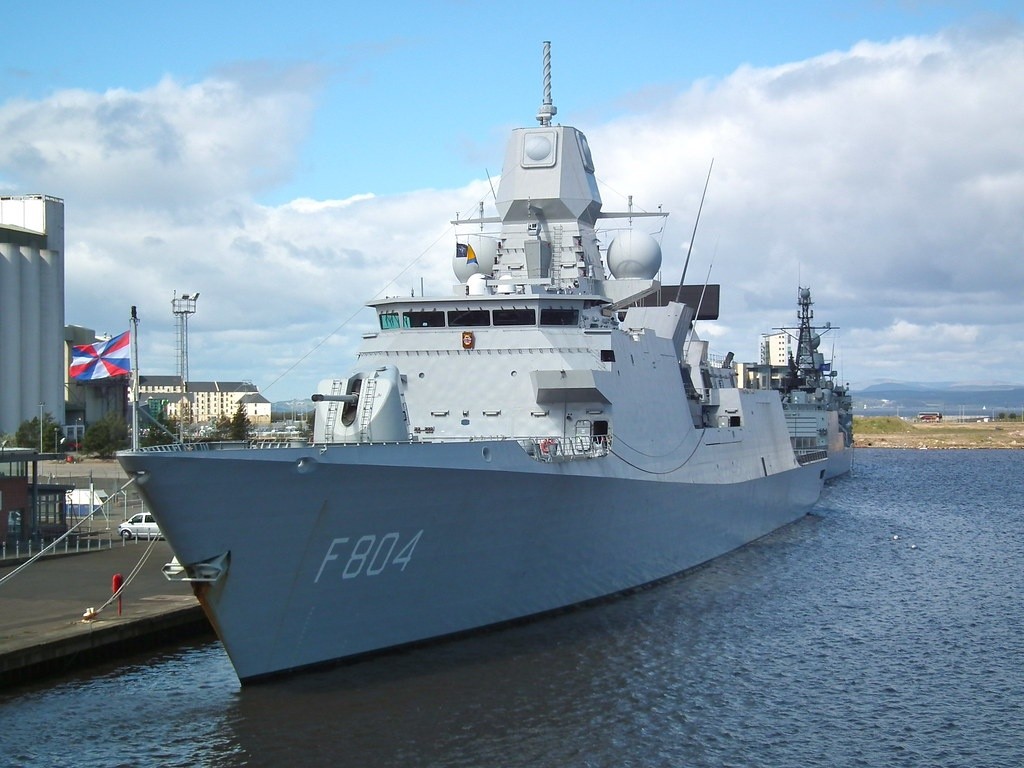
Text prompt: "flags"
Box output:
[[466, 244, 479, 269], [70, 329, 130, 380]]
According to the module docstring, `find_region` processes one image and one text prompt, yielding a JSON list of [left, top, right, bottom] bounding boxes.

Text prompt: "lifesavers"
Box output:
[[540, 438, 554, 454]]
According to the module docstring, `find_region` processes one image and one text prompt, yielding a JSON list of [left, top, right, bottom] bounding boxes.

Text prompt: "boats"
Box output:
[[709, 277, 857, 479], [112, 37, 828, 689]]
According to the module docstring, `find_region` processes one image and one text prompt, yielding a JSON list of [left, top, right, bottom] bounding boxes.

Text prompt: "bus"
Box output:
[[916, 411, 942, 420]]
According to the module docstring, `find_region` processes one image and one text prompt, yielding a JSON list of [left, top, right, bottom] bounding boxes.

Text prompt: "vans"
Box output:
[[919, 416, 941, 423], [117, 512, 166, 541]]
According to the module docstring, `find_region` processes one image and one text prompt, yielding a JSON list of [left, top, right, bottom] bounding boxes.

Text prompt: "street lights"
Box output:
[[37, 402, 47, 454]]
